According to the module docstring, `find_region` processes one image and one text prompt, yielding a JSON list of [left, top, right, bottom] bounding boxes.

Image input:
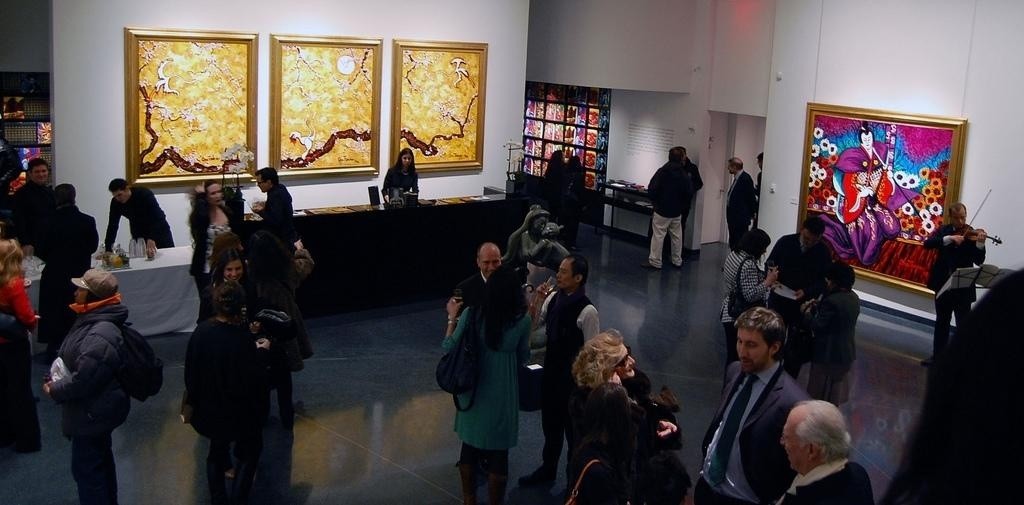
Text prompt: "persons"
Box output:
[[503, 204, 571, 285], [726, 153, 763, 251], [693, 307, 811, 502], [4, 146, 98, 453], [719, 217, 860, 407], [779, 400, 872, 502], [43, 267, 130, 499], [184, 167, 313, 504], [544, 150, 585, 246], [104, 179, 174, 264], [382, 148, 419, 203], [640, 147, 703, 270], [438, 242, 692, 502], [919, 204, 986, 367]]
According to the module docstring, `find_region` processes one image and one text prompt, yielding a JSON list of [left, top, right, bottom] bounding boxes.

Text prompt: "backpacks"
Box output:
[[110, 319, 164, 402]]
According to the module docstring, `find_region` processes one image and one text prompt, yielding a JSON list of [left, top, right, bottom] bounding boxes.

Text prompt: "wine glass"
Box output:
[[98, 240, 123, 269]]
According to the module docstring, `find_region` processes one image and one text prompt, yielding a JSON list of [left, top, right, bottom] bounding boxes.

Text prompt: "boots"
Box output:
[[486, 471, 509, 505], [206, 463, 257, 505], [458, 463, 480, 505]]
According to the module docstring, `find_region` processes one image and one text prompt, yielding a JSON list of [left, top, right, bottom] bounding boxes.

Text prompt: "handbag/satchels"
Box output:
[[0, 311, 26, 341], [727, 257, 757, 319], [434, 330, 483, 395]]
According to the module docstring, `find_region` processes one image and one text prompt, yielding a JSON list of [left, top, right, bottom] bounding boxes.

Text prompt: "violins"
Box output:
[[952, 224, 1003, 245]]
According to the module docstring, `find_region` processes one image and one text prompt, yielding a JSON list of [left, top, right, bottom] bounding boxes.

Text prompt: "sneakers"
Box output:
[[640, 260, 663, 270]]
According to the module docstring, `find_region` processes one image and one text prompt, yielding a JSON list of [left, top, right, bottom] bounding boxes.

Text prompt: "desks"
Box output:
[[597, 182, 654, 238], [28, 247, 211, 353]]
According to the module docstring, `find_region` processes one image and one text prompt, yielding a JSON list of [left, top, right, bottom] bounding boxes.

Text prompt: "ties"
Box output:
[[707, 372, 758, 488]]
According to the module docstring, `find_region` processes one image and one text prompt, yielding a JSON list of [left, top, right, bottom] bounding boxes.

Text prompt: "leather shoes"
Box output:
[[518, 469, 555, 487]]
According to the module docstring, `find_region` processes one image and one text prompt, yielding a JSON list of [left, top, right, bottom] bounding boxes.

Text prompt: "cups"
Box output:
[[540, 276, 556, 297], [129, 238, 146, 258], [453, 287, 463, 304]]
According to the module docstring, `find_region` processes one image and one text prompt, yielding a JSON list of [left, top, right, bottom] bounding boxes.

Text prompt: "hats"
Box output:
[[69, 267, 119, 300]]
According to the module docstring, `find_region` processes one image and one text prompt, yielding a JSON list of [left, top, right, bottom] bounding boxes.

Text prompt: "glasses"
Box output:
[[615, 345, 632, 368]]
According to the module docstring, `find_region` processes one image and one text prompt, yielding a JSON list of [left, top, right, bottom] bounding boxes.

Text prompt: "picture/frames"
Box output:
[[121, 25, 257, 192], [795, 102, 968, 300], [391, 39, 487, 175], [270, 32, 381, 180]]
